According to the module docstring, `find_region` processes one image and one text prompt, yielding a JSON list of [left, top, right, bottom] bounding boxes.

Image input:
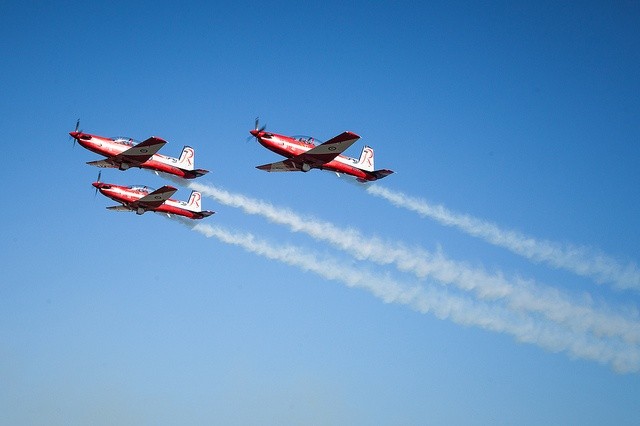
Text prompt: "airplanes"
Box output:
[[69, 118, 209, 179], [92, 170, 215, 219], [249, 119, 394, 183]]
